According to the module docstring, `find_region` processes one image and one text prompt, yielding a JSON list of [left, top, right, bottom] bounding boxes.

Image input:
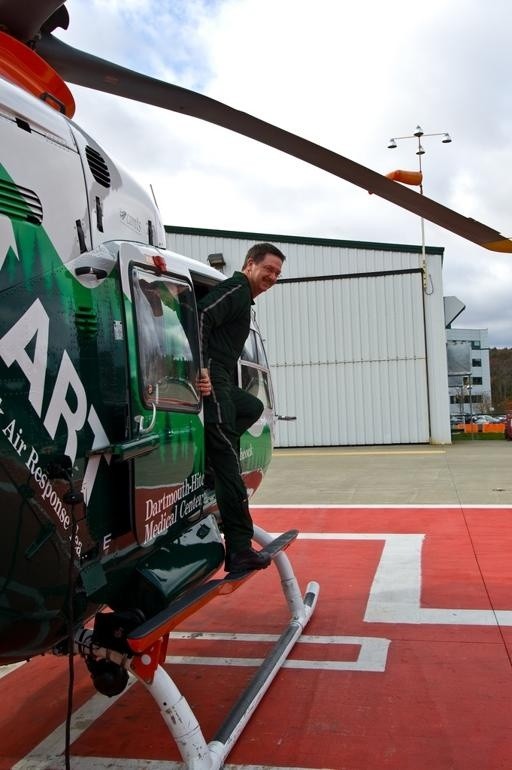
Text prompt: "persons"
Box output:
[[192, 242, 287, 573]]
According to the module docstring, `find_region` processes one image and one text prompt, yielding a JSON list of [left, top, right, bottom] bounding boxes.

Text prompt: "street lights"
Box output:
[[383, 124, 454, 291], [466, 384, 475, 439]]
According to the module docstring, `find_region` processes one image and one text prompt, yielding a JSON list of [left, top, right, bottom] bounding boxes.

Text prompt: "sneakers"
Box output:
[[224, 547, 272, 573]]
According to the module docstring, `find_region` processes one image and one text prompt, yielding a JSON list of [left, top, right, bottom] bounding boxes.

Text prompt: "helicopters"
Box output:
[[1, 1, 511, 770]]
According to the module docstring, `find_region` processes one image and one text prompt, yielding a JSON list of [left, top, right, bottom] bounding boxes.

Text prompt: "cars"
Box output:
[[449, 413, 511, 440]]
